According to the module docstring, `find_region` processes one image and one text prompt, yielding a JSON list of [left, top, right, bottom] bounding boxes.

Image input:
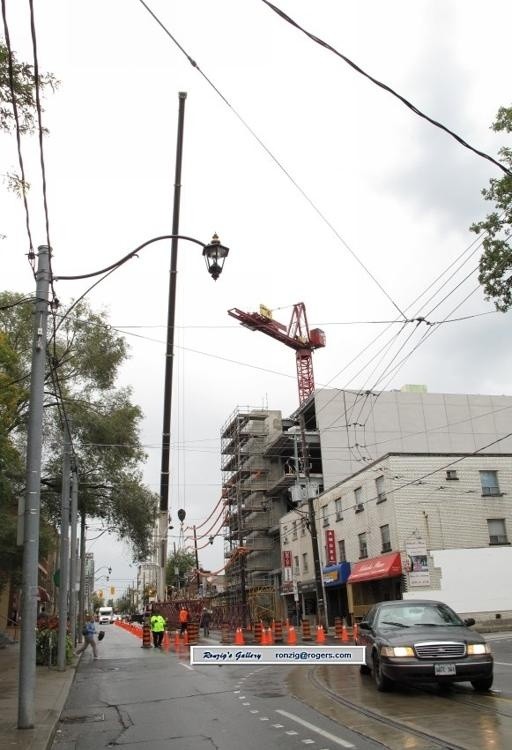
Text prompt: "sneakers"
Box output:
[[72, 651, 80, 657], [154, 644, 164, 649], [92, 656, 102, 660]]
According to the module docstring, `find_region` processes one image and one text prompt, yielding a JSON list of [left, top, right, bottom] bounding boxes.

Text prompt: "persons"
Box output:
[[151, 609, 168, 648], [179, 606, 189, 636], [73, 616, 99, 660], [201, 607, 211, 637]]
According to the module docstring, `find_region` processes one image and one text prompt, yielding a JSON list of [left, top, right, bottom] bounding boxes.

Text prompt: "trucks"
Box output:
[[96, 605, 113, 625]]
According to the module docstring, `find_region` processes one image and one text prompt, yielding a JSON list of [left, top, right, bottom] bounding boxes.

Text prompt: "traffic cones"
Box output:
[[232, 614, 360, 646], [162, 631, 169, 645], [114, 619, 143, 638], [173, 630, 181, 645], [182, 629, 189, 645]]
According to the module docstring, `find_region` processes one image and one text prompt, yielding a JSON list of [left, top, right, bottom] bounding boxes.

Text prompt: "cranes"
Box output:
[[227, 299, 327, 407]]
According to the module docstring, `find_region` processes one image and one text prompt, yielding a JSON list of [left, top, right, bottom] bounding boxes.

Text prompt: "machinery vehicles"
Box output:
[[134, 92, 187, 620]]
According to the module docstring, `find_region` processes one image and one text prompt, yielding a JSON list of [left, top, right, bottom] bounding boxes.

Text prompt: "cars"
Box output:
[[356, 597, 494, 695], [112, 615, 122, 622]]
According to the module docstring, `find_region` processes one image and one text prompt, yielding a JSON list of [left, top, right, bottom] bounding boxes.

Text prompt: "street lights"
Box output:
[[15, 230, 235, 730], [87, 567, 113, 611]]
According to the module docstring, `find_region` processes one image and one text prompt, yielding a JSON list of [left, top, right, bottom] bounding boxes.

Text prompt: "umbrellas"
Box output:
[[95, 631, 105, 640]]
